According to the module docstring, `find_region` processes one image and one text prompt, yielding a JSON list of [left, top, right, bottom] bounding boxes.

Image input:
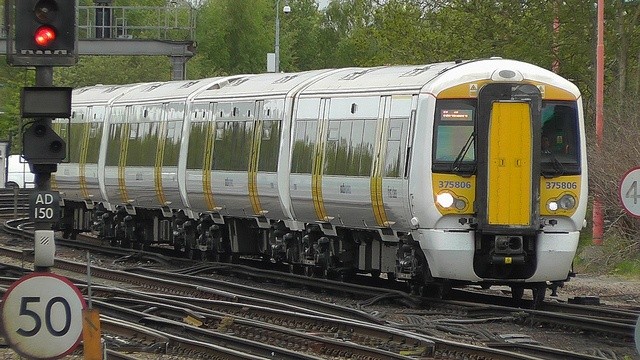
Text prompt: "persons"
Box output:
[[543, 105, 574, 153]]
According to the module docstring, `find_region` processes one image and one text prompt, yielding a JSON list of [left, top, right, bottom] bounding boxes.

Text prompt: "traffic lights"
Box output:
[[14, 0, 74, 51]]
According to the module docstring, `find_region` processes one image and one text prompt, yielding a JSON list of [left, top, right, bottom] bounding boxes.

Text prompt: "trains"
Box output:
[[51, 54, 591, 303]]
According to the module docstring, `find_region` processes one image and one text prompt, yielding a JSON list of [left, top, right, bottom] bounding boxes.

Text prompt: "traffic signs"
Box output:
[[618, 165, 640, 219], [0, 271, 88, 360]]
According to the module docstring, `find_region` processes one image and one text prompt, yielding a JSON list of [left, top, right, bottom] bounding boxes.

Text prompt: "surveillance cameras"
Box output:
[[283, 5, 291, 14]]
